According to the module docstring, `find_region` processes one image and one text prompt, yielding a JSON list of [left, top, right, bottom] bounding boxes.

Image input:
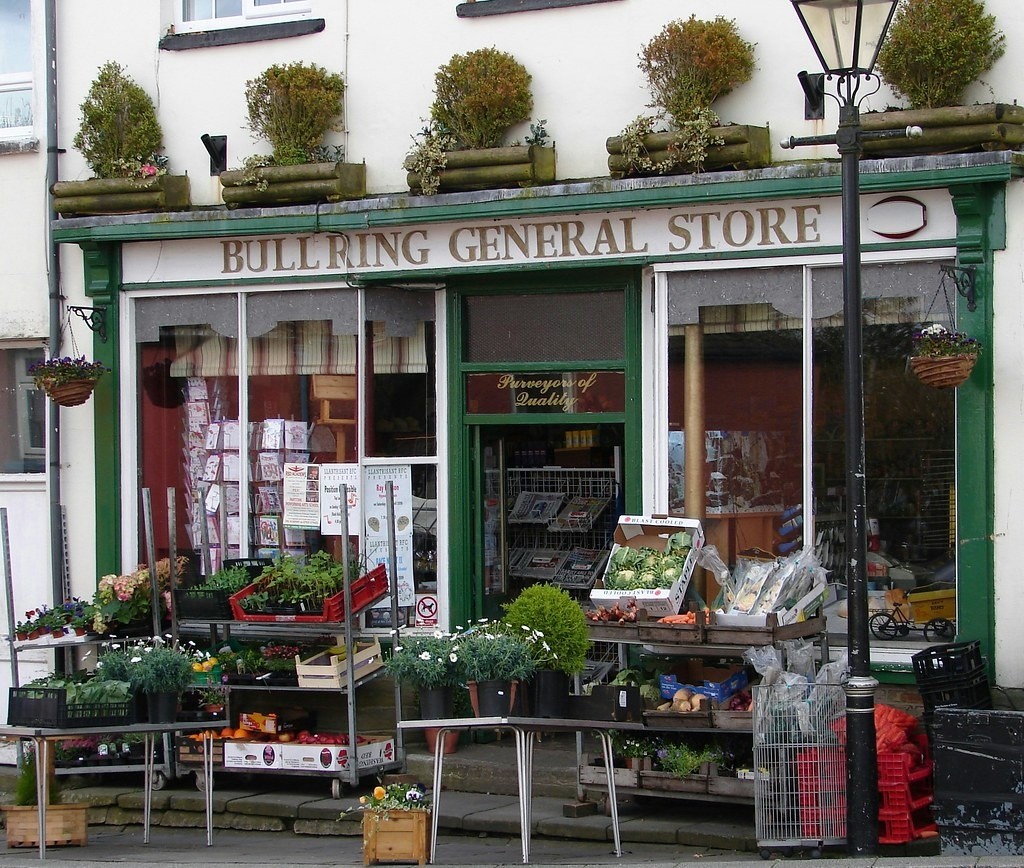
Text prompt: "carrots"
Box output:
[[656, 609, 709, 624], [834, 704, 916, 753]]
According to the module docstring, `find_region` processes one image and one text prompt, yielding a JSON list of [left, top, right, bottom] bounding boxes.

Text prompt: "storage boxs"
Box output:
[[294, 633, 384, 689], [710, 581, 830, 629], [6, 688, 137, 730], [590, 515, 706, 618], [794, 638, 991, 845], [189, 638, 230, 687], [176, 737, 395, 772], [639, 662, 758, 731], [173, 557, 274, 621], [238, 707, 318, 734]]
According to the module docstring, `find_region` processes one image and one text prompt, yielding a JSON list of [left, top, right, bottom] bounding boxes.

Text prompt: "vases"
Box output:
[[417, 683, 456, 720], [425, 728, 460, 753], [626, 757, 645, 769], [910, 355, 977, 387], [17, 626, 63, 641], [126, 689, 177, 724], [466, 678, 519, 717], [572, 684, 660, 724], [75, 628, 85, 636], [205, 704, 224, 714], [363, 809, 432, 866], [46, 377, 98, 406], [66, 616, 72, 623], [55, 750, 164, 766]]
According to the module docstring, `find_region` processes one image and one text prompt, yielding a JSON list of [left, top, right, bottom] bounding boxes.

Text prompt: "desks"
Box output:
[[0, 719, 230, 859], [396, 716, 644, 864]]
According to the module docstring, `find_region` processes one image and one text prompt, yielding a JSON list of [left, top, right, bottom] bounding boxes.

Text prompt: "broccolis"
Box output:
[[606, 531, 689, 590]]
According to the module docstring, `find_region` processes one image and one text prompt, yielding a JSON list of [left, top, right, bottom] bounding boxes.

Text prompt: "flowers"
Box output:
[[83, 633, 211, 692], [17, 596, 89, 634], [27, 355, 111, 378], [196, 675, 232, 705], [60, 735, 104, 757], [368, 619, 464, 688], [138, 164, 156, 176], [90, 556, 190, 635], [336, 782, 433, 822], [618, 731, 738, 761], [912, 324, 977, 356], [450, 618, 558, 679], [262, 645, 300, 664]]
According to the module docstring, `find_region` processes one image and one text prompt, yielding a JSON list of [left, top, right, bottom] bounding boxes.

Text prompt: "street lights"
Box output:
[[789, 1, 897, 858]]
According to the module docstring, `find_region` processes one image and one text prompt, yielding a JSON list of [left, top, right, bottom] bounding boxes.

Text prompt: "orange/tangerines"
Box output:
[[188, 728, 250, 739]]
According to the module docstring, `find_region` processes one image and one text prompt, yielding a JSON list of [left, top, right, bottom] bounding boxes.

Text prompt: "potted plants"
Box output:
[[220, 59, 366, 210], [0, 756, 89, 847], [858, 0, 1024, 160], [589, 729, 619, 765], [500, 581, 595, 719], [404, 44, 556, 194], [238, 550, 376, 615], [606, 14, 772, 179], [49, 59, 190, 217]]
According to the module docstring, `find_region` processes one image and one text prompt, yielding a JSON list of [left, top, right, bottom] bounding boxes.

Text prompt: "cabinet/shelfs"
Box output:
[[483, 446, 622, 589], [573, 630, 831, 803], [669, 437, 955, 608], [167, 482, 405, 784]]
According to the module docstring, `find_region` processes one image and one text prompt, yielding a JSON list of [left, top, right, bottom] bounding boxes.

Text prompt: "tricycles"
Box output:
[[867, 588, 955, 645]]
